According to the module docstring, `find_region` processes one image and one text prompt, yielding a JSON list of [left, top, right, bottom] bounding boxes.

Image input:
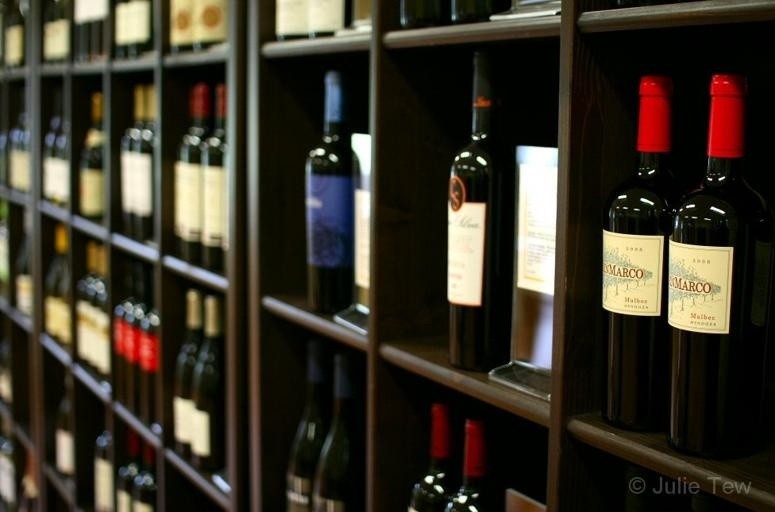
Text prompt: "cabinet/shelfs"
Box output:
[[240, 0, 775, 512], [0, 1, 246, 512]]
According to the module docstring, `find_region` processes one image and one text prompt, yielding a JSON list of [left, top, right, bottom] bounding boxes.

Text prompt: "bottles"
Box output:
[[93, 424, 158, 512], [445, 47, 515, 371], [112, 83, 156, 240], [15, 204, 36, 318], [67, 240, 113, 382], [274, 0, 375, 42], [54, 373, 79, 478], [5, 105, 29, 193], [0, 198, 10, 301], [167, 0, 230, 54], [402, 403, 452, 512], [597, 71, 679, 434], [72, 0, 109, 64], [167, 78, 212, 267], [37, 97, 68, 210], [313, 354, 368, 512], [42, 0, 71, 63], [107, 256, 162, 433], [301, 69, 359, 314], [663, 64, 769, 463], [194, 85, 232, 271], [446, 417, 494, 512], [281, 341, 330, 512], [39, 222, 74, 351], [168, 286, 200, 460], [72, 91, 102, 220], [113, 0, 154, 60], [0, 429, 18, 509], [0, 316, 16, 411], [3, 0, 26, 68], [183, 295, 231, 473]]
